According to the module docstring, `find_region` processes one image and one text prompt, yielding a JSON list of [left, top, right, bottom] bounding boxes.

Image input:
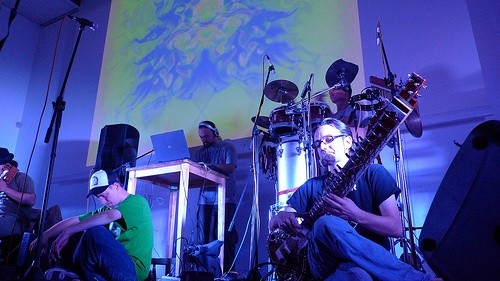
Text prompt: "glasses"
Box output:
[[311, 134, 348, 149]]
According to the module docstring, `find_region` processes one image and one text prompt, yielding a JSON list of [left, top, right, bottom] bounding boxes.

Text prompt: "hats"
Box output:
[[86, 169, 121, 199], [0, 147, 14, 165]]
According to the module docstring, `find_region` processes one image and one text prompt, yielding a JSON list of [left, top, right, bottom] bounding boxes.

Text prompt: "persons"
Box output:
[[326, 82, 365, 130], [0, 146, 37, 281], [186, 120, 240, 281], [268, 116, 447, 281], [27, 167, 155, 281]]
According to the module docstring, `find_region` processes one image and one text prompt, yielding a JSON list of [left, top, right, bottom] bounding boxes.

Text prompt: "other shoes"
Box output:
[[44, 268, 80, 281]]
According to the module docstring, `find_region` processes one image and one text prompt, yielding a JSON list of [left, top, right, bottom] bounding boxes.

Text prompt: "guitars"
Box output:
[[266, 72, 427, 281]]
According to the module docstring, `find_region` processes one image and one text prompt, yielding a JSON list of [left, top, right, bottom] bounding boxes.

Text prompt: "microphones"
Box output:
[[267, 56, 276, 74], [300, 75, 311, 98], [376, 26, 381, 46], [68, 15, 98, 30]]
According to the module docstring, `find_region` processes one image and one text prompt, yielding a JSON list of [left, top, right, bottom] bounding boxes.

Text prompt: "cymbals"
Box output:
[[251, 115, 270, 129], [263, 79, 299, 103], [404, 110, 423, 138], [324, 58, 360, 86], [349, 93, 383, 111]]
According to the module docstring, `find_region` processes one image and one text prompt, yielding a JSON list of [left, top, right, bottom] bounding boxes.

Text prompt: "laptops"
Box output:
[[150, 129, 192, 163]]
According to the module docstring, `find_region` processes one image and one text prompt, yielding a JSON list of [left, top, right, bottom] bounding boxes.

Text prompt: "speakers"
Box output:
[[93, 123, 140, 183]]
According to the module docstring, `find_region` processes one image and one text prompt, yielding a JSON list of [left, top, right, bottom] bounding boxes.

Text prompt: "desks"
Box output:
[[125, 159, 231, 277]]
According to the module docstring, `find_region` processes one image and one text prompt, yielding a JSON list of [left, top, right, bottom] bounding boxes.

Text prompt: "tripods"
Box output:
[[389, 136, 427, 272]]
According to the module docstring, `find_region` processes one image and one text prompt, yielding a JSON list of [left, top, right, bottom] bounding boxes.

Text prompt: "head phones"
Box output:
[[199, 123, 219, 137]]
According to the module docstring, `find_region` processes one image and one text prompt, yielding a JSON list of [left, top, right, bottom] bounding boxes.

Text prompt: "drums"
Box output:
[[304, 102, 331, 126], [275, 134, 316, 213], [269, 106, 304, 138]]
